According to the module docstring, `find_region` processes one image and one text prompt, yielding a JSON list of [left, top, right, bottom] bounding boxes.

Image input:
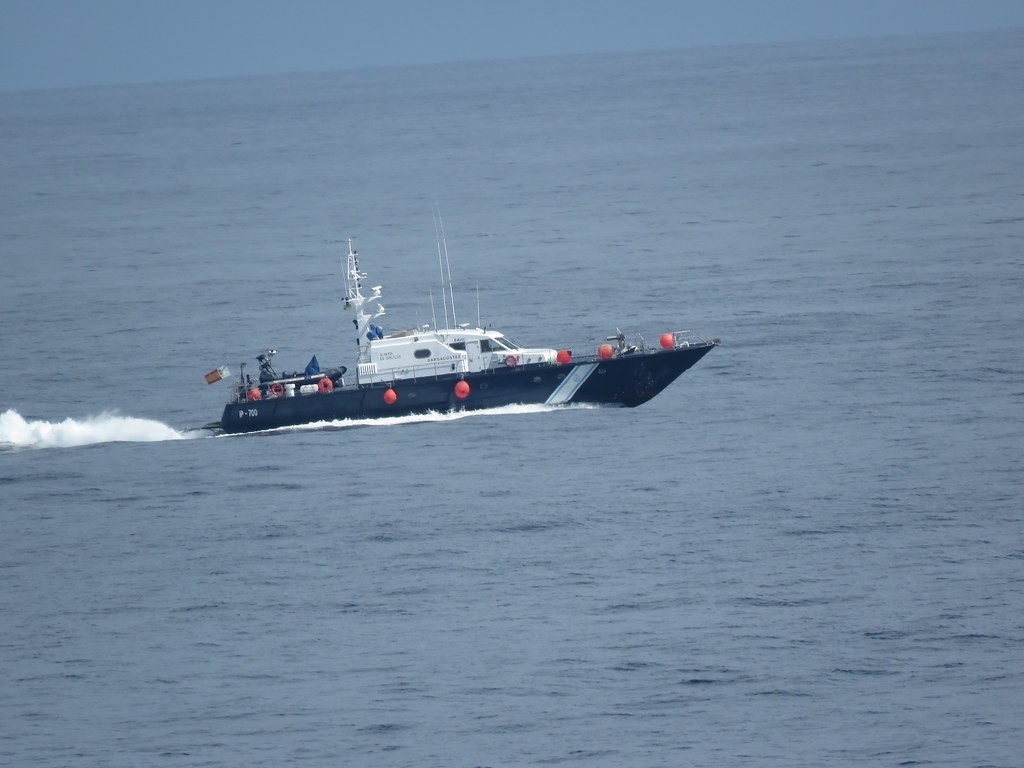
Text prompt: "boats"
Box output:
[[200, 238, 724, 439]]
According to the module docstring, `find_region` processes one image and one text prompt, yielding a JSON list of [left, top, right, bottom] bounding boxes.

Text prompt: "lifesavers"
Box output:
[[317, 378, 332, 392], [504, 355, 517, 369], [271, 383, 284, 397]]
